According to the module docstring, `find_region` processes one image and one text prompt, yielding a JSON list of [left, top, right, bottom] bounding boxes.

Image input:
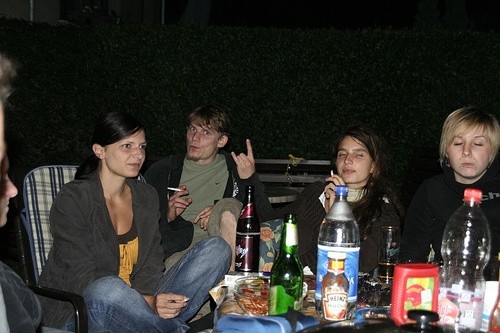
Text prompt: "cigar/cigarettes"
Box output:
[[331, 169, 334, 177], [167, 187, 185, 191], [180, 297, 190, 302]]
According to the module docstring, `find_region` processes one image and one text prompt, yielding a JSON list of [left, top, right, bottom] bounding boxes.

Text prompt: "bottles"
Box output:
[[315, 185, 360, 319], [235, 185, 260, 273], [488, 252, 500, 333], [320, 252, 349, 325], [434, 188, 491, 333], [390, 263, 439, 326], [268, 213, 304, 316]]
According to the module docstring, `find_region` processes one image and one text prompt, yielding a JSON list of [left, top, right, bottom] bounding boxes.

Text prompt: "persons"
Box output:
[[401, 106, 500, 282], [0, 54, 40, 333], [36, 109, 233, 333], [231, 121, 403, 274], [144, 101, 242, 314]]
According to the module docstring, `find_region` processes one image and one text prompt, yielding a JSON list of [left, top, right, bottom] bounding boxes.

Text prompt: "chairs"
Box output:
[[21, 164, 88, 333]]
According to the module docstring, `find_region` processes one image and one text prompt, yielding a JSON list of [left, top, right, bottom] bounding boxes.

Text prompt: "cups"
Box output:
[[378, 226, 400, 279]]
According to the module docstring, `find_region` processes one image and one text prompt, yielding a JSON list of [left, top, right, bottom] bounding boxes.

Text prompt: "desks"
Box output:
[[264, 187, 299, 204]]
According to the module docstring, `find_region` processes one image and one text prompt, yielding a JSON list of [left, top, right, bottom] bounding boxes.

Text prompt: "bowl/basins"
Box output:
[[234, 276, 307, 316], [298, 319, 444, 333]]
